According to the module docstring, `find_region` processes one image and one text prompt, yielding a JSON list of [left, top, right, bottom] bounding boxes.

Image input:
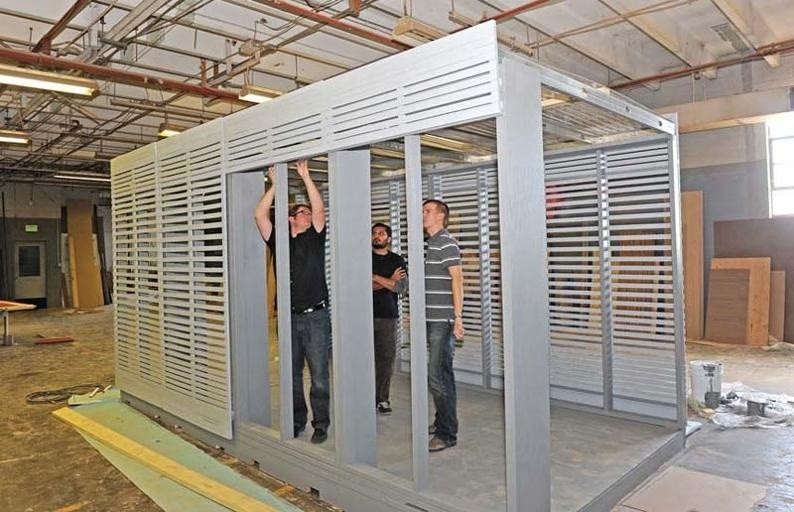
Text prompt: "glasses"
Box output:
[[293, 209, 311, 215]]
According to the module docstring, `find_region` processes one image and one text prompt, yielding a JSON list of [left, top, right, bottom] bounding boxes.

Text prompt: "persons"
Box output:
[[254, 160, 330, 444], [372, 223, 406, 416], [423, 199, 466, 453]]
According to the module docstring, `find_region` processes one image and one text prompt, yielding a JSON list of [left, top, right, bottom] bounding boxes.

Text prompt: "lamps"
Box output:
[[157, 110, 192, 136], [240, 70, 286, 104], [1, 27, 100, 98], [1, 108, 32, 145]]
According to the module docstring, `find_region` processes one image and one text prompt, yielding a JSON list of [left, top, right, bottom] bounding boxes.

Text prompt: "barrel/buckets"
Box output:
[[689, 358, 721, 404]]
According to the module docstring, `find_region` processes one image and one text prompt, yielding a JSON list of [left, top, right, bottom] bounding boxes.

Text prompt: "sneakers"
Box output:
[[311, 428, 328, 443], [377, 402, 393, 414], [429, 424, 456, 452]]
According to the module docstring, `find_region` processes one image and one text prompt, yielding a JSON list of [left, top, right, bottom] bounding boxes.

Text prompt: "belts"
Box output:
[[292, 300, 327, 314]]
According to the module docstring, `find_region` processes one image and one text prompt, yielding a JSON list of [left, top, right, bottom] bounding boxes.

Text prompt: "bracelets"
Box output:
[[454, 313, 463, 319]]
